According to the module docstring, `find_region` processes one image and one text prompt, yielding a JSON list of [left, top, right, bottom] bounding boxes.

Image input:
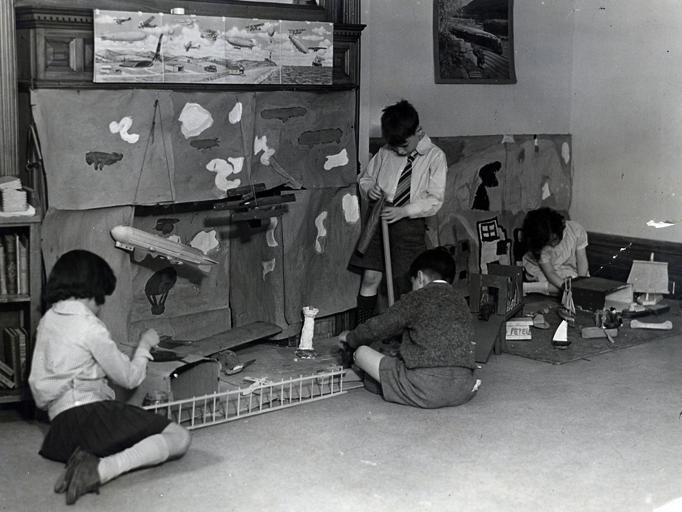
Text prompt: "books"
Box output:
[[0, 231, 29, 390]]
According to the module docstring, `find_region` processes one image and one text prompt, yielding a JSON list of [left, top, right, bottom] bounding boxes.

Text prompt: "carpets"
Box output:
[[501, 292, 681, 365]]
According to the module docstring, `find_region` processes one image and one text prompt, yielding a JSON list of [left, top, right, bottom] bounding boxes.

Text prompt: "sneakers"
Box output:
[[55, 446, 99, 504]]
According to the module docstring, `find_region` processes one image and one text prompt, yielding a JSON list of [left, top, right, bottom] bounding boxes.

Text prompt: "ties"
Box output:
[[394, 153, 418, 207]]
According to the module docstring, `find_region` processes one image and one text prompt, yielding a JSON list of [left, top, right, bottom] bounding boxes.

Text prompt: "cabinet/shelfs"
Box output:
[[0, 187, 43, 419]]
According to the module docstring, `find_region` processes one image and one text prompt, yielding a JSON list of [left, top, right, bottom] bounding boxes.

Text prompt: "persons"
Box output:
[[336, 246, 482, 410], [26, 249, 191, 505], [518, 206, 589, 296], [348, 99, 449, 327]]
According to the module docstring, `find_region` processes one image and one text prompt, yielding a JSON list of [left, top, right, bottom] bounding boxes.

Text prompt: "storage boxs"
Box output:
[[109, 340, 221, 413]]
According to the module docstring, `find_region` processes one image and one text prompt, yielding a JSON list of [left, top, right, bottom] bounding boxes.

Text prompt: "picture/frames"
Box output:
[[433, 0, 518, 85]]
